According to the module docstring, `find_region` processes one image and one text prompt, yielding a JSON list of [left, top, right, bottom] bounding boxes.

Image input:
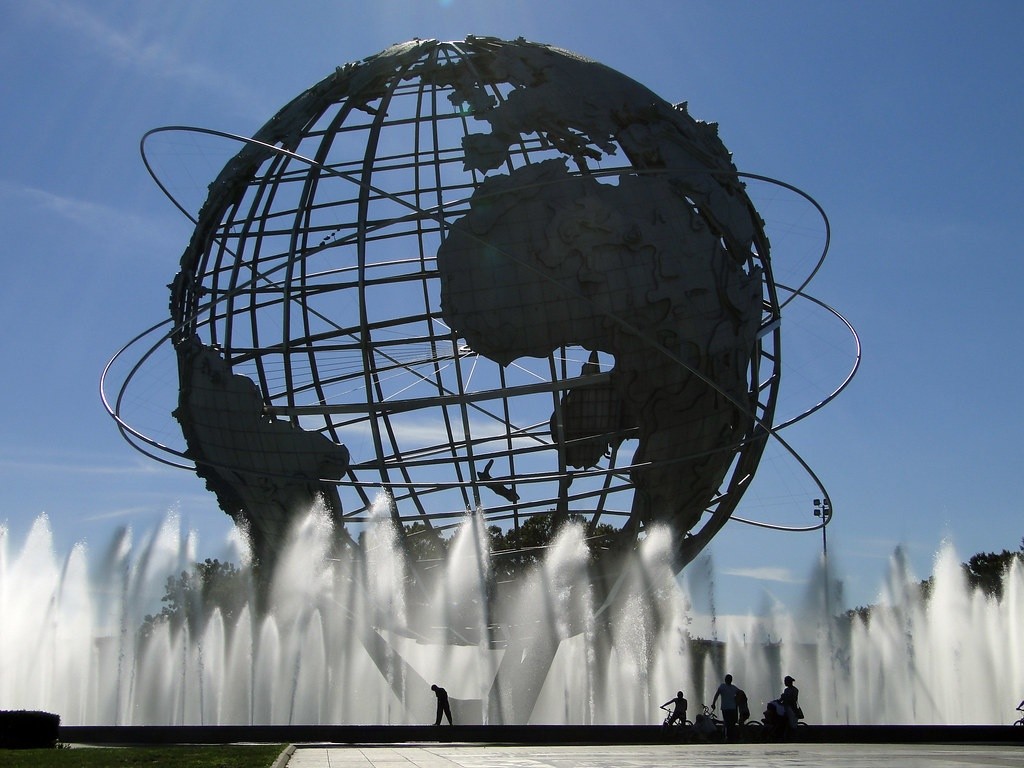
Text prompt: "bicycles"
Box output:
[[698, 703, 764, 742], [758, 707, 811, 742], [1013, 707, 1024, 729], [660, 706, 695, 741]]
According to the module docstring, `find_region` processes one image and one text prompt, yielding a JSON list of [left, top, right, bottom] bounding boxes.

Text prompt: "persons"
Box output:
[[659, 690, 688, 725], [711, 674, 741, 724], [736, 689, 750, 725], [780, 675, 798, 725], [430, 684, 453, 726], [1016, 700, 1024, 709]]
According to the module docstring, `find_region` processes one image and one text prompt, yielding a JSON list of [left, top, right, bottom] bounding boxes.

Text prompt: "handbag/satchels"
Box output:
[[797, 707, 804, 719]]
